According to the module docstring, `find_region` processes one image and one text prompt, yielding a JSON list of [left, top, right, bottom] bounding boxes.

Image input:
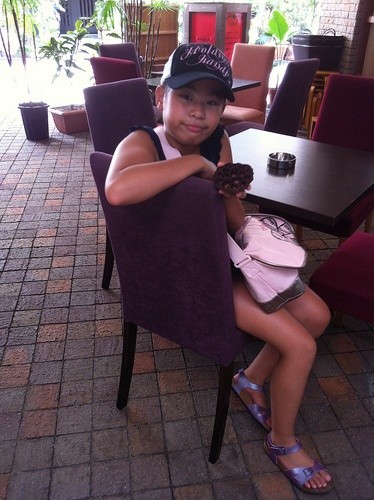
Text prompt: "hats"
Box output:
[[160, 44, 236, 103]]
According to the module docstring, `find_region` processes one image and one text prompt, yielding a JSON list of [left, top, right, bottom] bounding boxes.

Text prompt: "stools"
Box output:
[[305, 70, 341, 129]]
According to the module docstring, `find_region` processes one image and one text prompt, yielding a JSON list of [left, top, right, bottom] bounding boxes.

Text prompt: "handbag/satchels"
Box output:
[[230, 213, 307, 313]]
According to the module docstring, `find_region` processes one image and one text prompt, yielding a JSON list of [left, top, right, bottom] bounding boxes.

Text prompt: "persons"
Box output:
[[104, 43, 334, 494]]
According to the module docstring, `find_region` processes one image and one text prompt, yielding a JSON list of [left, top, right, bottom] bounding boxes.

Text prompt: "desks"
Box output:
[[146, 77, 261, 92], [228, 128, 374, 227]]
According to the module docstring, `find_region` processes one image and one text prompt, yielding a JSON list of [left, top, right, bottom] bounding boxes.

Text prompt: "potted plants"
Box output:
[[0, 0, 127, 141], [263, 8, 312, 107]]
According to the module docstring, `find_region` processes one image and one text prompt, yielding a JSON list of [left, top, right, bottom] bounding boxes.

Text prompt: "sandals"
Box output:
[[262, 432, 335, 494], [232, 369, 271, 431]]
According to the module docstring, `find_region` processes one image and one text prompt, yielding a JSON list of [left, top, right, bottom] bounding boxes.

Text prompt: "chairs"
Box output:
[[220, 43, 275, 125], [99, 43, 144, 78], [90, 57, 163, 123], [89, 153, 257, 464], [310, 231, 374, 326], [311, 73, 374, 247], [84, 77, 158, 289], [226, 58, 320, 138]]
